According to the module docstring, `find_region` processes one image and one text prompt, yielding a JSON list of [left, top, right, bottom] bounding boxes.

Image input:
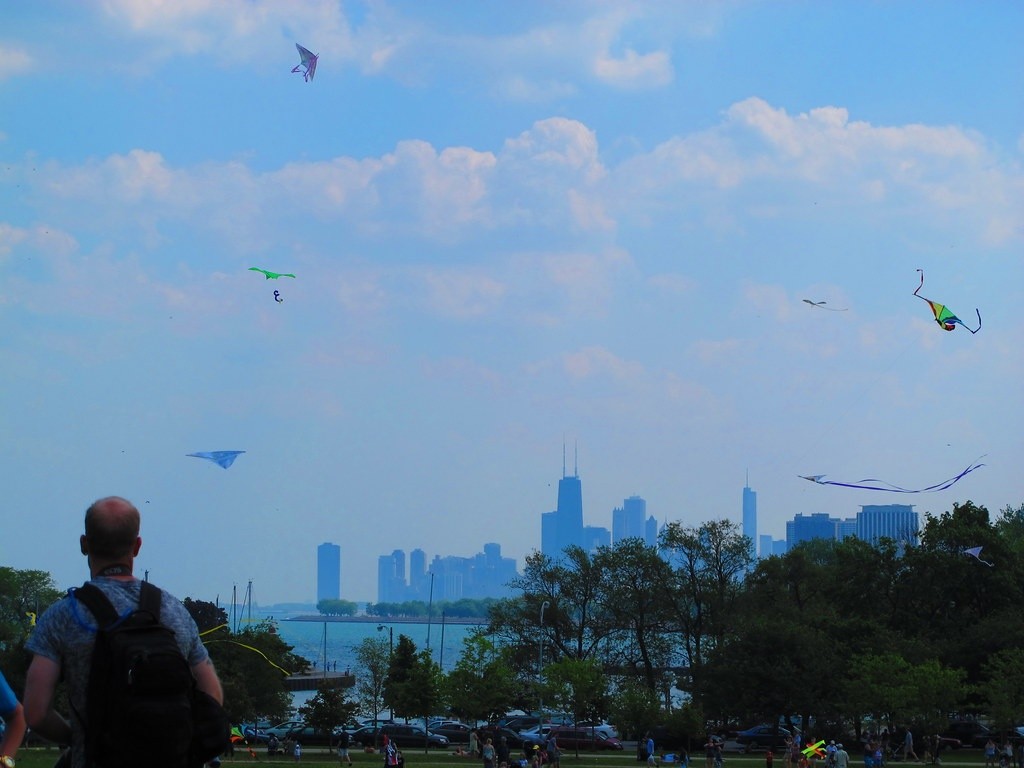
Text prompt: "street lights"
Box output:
[[539, 602, 550, 741], [377, 623, 393, 720]]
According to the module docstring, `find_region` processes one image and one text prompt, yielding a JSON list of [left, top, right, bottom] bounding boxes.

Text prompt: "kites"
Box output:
[[798, 454, 987, 493], [230, 725, 262, 764], [185, 450, 247, 470], [803, 299, 848, 312], [801, 740, 827, 760], [961, 546, 994, 567], [291, 43, 320, 82], [912, 269, 981, 334], [248, 267, 296, 304]]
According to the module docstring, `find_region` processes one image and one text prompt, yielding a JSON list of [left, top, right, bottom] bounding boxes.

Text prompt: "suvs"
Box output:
[[544, 726, 623, 750], [377, 724, 450, 749]]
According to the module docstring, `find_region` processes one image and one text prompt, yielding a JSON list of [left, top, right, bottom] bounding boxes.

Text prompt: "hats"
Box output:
[[533, 745, 539, 749]]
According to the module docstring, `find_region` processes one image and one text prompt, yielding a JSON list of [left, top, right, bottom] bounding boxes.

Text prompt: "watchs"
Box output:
[[0, 754, 15, 768]]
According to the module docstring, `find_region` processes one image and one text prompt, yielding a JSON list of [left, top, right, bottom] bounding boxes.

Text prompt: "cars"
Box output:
[[265, 721, 356, 747], [702, 725, 809, 754], [233, 710, 619, 744], [922, 722, 1024, 752]]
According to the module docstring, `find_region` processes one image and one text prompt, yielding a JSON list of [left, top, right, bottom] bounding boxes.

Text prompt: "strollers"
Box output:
[[887, 742, 903, 761]]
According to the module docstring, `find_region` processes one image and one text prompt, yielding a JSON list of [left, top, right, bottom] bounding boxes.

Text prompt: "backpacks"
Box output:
[[68, 581, 231, 768]]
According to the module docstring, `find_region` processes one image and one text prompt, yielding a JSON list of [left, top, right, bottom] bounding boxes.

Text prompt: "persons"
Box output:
[[881, 729, 904, 761], [333, 726, 352, 767], [0, 672, 27, 768], [682, 659, 686, 667], [452, 726, 565, 768], [766, 729, 887, 768], [313, 661, 351, 674], [900, 726, 1024, 768], [638, 733, 727, 768], [563, 716, 567, 724], [294, 741, 302, 762], [267, 735, 279, 754], [23, 496, 224, 768], [380, 732, 405, 768]]
